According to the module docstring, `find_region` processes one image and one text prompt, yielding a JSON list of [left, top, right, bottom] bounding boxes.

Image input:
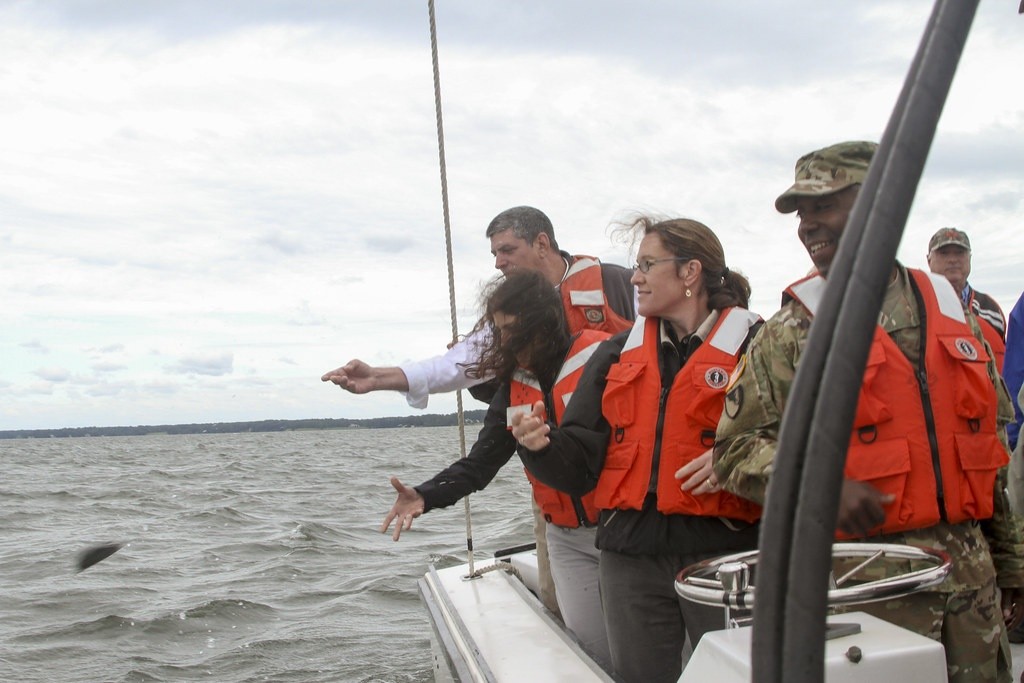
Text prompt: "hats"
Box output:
[[775, 140, 879, 214], [928, 228, 972, 255]]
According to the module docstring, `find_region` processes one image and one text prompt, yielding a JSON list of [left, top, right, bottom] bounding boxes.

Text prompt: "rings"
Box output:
[[707, 479, 714, 489], [522, 437, 524, 444]]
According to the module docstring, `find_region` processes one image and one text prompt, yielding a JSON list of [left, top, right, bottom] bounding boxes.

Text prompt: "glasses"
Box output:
[[629, 257, 692, 274]]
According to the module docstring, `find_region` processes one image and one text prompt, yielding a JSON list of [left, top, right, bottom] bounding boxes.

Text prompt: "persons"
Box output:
[[1002, 290, 1024, 516], [320, 204, 639, 622], [512, 217, 765, 683], [712, 141, 1024, 683], [927, 228, 1006, 375], [381, 267, 614, 671]]
